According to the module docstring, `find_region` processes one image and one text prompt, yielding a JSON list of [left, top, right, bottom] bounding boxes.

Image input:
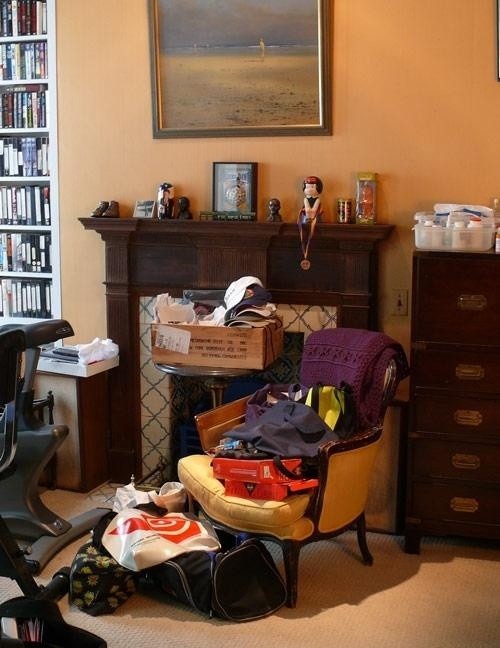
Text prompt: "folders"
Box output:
[[12, 279, 50, 318]]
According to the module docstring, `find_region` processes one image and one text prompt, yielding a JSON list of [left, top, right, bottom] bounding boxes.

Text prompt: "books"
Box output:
[[0, 232, 52, 273], [0, 0, 47, 36], [0, 42, 48, 80], [0, 184, 51, 226], [0, 278, 52, 319], [0, 84, 47, 128], [0, 136, 50, 177]]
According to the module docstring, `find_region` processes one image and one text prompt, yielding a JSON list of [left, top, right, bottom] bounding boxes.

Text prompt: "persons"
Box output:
[[160, 190, 174, 217], [266, 198, 281, 221], [176, 197, 191, 219], [259, 37, 265, 58]]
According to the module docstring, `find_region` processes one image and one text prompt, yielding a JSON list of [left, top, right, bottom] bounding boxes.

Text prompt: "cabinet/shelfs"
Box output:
[[406, 247, 500, 554], [0, 0, 62, 350], [33, 370, 109, 493]]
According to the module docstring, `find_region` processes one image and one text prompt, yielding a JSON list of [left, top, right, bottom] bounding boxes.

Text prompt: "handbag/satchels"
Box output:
[[102, 509, 222, 573], [136, 527, 288, 623], [69, 543, 136, 616], [112, 479, 186, 514], [212, 378, 353, 481]]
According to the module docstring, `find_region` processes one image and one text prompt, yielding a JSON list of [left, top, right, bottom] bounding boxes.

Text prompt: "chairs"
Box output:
[[174, 326, 410, 610]]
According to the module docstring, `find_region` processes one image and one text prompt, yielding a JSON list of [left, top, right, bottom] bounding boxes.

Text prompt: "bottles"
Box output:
[[492, 198, 500, 253], [207, 440, 243, 452], [356, 171, 378, 225], [423, 218, 483, 250]]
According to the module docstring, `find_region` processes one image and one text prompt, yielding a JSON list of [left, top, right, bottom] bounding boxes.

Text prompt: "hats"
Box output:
[[223, 276, 277, 329]]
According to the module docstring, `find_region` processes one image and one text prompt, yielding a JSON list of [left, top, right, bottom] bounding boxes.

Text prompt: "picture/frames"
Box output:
[[212, 162, 258, 219], [148, 0, 333, 139]]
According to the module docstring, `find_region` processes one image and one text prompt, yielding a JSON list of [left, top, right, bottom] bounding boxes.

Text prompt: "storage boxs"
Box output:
[[411, 212, 500, 252], [150, 314, 285, 370]]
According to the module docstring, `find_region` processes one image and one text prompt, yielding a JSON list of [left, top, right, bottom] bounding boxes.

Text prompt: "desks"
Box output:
[[154, 357, 281, 408]]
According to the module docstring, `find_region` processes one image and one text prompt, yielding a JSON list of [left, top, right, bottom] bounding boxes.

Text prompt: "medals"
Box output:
[[300, 259, 311, 271]]
